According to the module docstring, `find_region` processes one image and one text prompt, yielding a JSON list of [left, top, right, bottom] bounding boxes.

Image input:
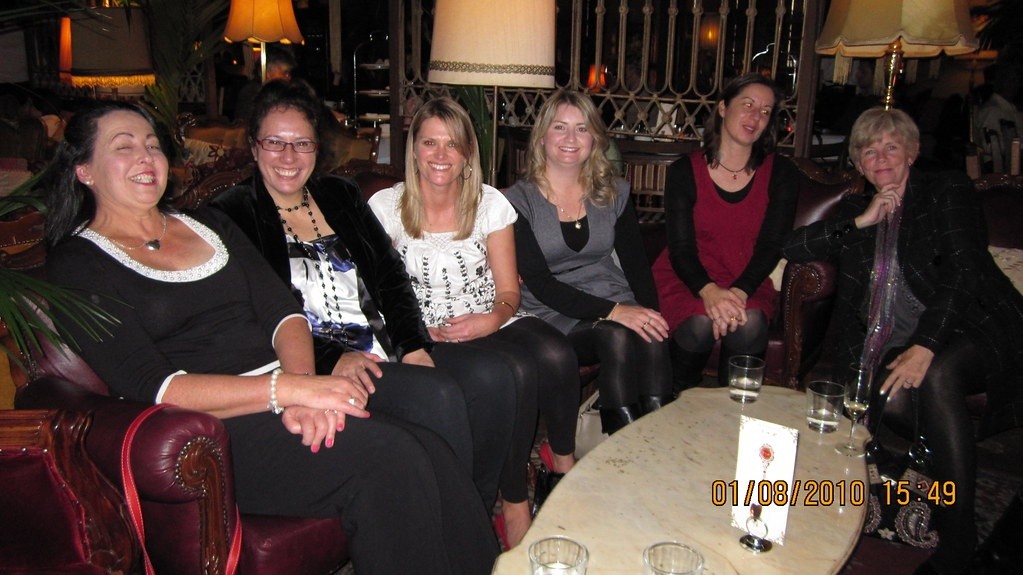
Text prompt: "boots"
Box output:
[[717, 342, 767, 389], [668, 335, 710, 397]]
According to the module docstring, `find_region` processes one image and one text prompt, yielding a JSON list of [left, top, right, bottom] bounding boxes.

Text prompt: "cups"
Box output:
[[729, 356, 767, 405], [806, 380, 847, 436], [528, 535, 590, 575], [644, 540, 705, 575]]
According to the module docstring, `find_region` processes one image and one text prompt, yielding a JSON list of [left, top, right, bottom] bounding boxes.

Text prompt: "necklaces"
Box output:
[[542, 187, 582, 229], [719, 162, 746, 180], [111, 211, 167, 250], [276, 187, 347, 347]]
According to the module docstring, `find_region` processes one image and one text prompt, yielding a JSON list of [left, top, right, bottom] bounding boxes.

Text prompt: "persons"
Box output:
[[651, 75, 803, 388], [504, 92, 670, 437], [45, 98, 500, 575], [785, 109, 1023, 575], [368, 96, 580, 548], [206, 96, 516, 517], [240, 40, 1023, 175], [0, 69, 91, 160]]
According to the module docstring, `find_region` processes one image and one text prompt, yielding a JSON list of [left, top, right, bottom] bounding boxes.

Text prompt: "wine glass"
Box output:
[[833, 363, 873, 457]]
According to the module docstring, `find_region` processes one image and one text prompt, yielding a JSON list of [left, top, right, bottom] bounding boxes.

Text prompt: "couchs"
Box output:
[[1, 142, 863, 573]]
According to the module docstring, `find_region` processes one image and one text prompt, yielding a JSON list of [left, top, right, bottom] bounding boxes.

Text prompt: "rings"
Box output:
[[324, 409, 337, 414], [643, 322, 649, 329], [350, 397, 356, 404], [730, 316, 737, 320], [906, 380, 912, 385], [714, 316, 721, 322], [458, 338, 460, 343]]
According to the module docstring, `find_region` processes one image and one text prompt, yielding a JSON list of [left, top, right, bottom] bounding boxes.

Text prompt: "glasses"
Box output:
[[252, 132, 319, 153]]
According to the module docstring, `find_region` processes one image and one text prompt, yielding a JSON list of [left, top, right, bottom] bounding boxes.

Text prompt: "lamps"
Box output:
[[428, 0, 556, 185], [55, 0, 157, 106], [814, 0, 981, 112], [222, 0, 306, 82]]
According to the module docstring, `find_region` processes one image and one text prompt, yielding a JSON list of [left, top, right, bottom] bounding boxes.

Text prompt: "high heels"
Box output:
[[494, 510, 513, 550], [538, 441, 556, 475]]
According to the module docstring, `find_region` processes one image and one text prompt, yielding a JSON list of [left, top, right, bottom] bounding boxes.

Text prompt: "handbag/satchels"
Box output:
[[866, 376, 940, 549]]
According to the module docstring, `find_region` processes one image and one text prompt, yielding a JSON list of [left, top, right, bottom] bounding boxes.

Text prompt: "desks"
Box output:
[[491, 383, 868, 575]]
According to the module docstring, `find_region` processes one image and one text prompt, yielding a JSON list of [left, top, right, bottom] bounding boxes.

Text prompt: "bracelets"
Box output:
[[493, 301, 515, 316], [270, 369, 284, 414], [610, 303, 619, 321]]
